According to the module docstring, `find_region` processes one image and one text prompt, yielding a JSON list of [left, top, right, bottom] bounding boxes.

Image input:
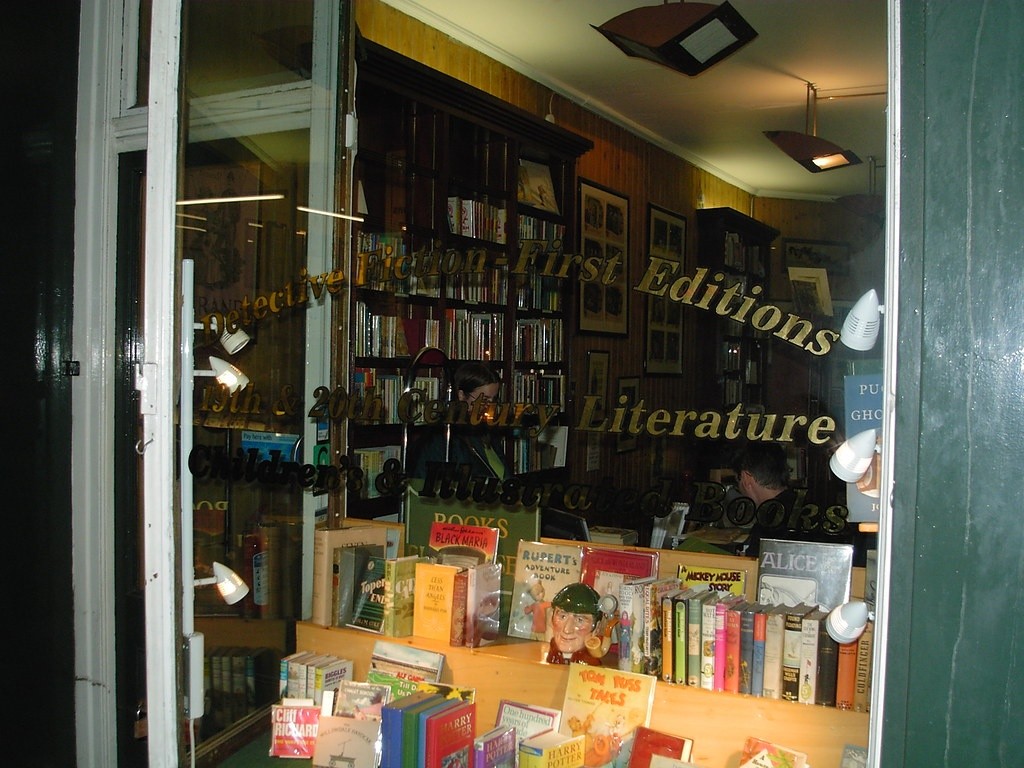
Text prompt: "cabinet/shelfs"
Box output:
[[697, 207, 780, 478], [348, 40, 596, 517]]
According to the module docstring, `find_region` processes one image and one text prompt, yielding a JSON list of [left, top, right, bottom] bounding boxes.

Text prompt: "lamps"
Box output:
[[193, 356, 249, 394], [824, 601, 874, 647], [829, 429, 882, 483], [193, 313, 250, 355], [762, 82, 863, 174], [194, 560, 250, 605], [840, 289, 885, 351], [589, 0, 760, 78]]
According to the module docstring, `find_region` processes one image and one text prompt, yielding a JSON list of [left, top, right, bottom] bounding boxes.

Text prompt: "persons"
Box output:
[[737, 440, 804, 559], [547, 582, 603, 666], [403, 363, 520, 509]]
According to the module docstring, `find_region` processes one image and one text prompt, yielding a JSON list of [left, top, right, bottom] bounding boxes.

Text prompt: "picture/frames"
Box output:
[[781, 235, 851, 281], [642, 202, 687, 378], [574, 175, 630, 337], [586, 349, 610, 420], [613, 375, 640, 454]]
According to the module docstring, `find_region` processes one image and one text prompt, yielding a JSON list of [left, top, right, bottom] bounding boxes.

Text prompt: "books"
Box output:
[[313, 525, 501, 651], [447, 196, 506, 243], [268, 640, 866, 768], [204, 648, 271, 732], [517, 214, 565, 255], [354, 231, 567, 501], [580, 547, 660, 658], [617, 538, 875, 714], [589, 525, 638, 548], [507, 540, 582, 642]]
